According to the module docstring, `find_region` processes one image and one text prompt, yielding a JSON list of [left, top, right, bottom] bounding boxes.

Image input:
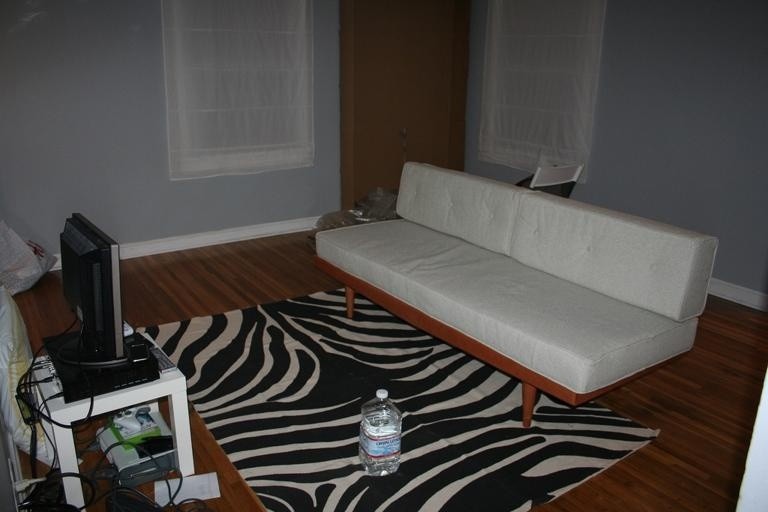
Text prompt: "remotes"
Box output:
[[148, 347, 177, 374], [123, 320, 135, 337]]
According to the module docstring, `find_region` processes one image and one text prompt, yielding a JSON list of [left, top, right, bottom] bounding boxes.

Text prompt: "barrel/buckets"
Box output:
[[358, 388, 403, 477]]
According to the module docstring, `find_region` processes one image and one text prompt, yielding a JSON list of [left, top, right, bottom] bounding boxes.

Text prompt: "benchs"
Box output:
[[316, 161, 718, 426]]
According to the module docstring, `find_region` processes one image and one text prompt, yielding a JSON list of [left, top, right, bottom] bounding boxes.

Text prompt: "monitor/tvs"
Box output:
[[60, 213, 128, 366]]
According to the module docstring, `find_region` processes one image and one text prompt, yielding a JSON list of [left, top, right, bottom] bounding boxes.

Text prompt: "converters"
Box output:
[[14, 392, 41, 425]]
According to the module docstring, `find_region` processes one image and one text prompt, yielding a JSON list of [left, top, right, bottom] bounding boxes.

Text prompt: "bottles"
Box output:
[[358, 388, 403, 477]]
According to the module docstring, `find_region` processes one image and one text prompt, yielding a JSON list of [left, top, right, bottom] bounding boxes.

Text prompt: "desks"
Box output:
[[29, 332, 194, 512]]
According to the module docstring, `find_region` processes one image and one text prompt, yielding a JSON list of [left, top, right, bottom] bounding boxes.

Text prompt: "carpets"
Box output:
[[137, 288, 660, 512]]
